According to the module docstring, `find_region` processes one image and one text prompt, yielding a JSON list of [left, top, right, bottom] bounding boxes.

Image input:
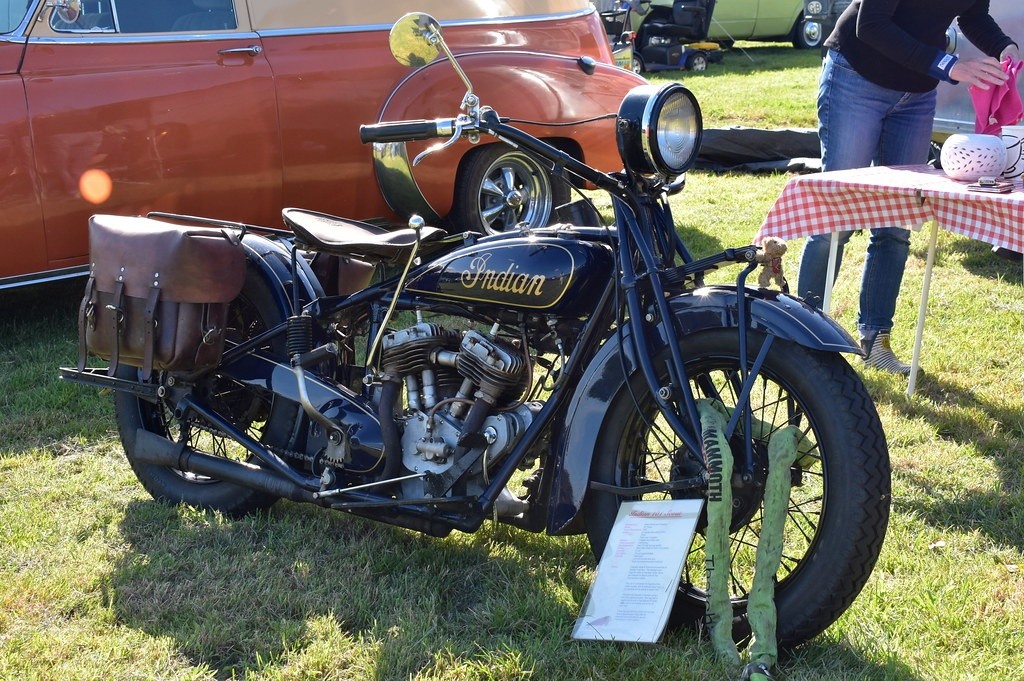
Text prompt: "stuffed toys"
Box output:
[[755, 236, 790, 293]]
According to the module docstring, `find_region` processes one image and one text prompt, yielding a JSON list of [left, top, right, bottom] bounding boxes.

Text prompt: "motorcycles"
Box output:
[[60, 10, 895, 650]]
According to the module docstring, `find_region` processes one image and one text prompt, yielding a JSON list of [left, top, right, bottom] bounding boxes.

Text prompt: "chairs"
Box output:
[[642, 1, 706, 35]]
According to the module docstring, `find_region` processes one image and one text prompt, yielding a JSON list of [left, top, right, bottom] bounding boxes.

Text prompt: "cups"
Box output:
[[1001, 125, 1024, 182]]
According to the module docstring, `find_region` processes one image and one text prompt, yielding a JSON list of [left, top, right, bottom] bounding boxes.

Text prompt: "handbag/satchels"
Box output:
[[275, 242, 376, 297], [77, 214, 246, 380]]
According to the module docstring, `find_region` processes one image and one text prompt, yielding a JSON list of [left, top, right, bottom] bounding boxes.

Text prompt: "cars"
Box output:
[[0, 0, 652, 293], [622, 0, 824, 53]]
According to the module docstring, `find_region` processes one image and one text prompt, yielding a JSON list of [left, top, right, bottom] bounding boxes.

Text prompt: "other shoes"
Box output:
[[853, 329, 925, 380]]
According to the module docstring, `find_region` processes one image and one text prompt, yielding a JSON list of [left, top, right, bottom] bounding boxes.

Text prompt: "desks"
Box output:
[[754, 164, 1024, 402]]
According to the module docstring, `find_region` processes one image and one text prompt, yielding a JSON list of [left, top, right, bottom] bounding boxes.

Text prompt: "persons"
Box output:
[[798, 0, 1021, 379]]
